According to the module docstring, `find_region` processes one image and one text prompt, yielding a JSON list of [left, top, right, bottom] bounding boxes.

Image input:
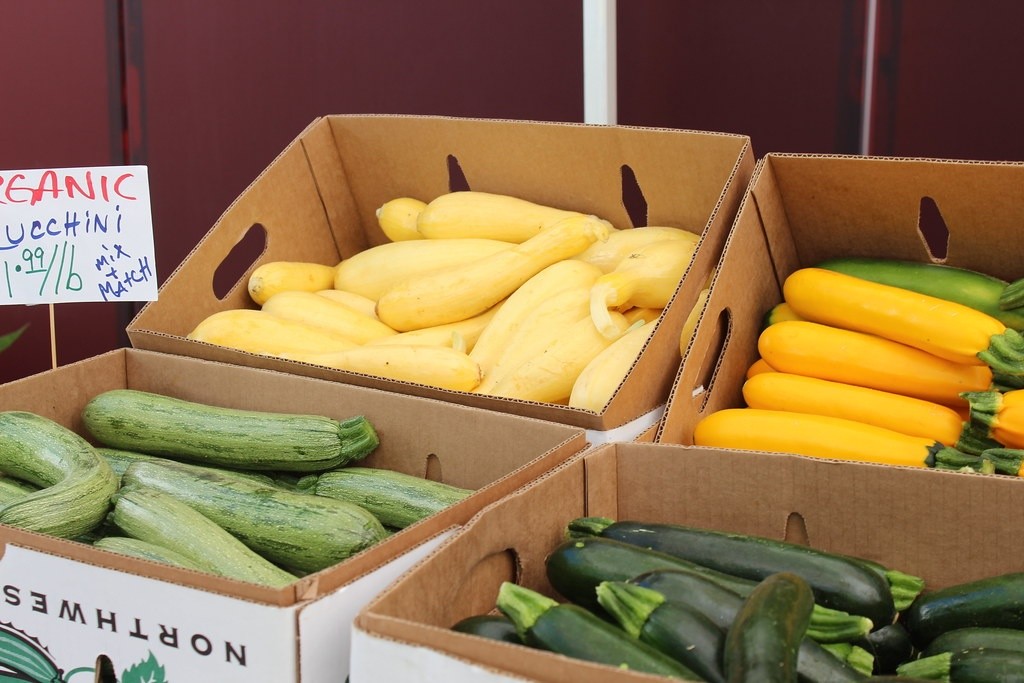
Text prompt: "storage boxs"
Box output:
[[125, 113, 756, 442], [1, 347, 593, 682], [653, 152, 1023, 446], [346, 441, 1023, 683]]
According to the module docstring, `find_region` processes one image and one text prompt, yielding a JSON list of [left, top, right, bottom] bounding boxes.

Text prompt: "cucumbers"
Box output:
[[449, 513, 1024, 683]]
